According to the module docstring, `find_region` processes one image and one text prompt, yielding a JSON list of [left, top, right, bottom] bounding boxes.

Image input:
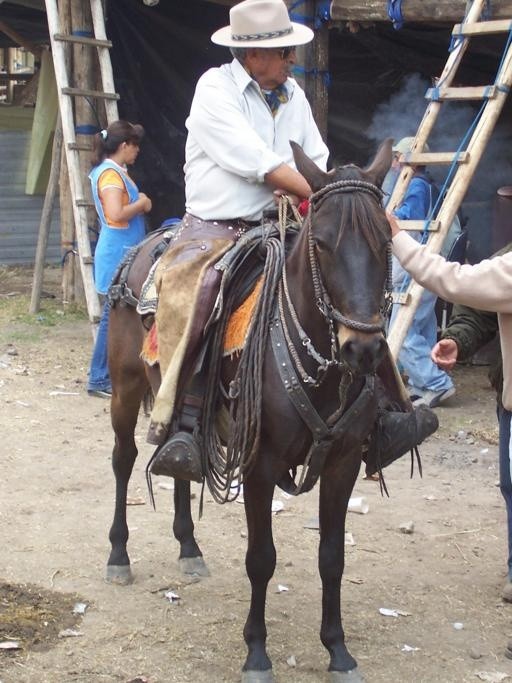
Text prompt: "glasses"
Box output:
[[269, 47, 297, 59]]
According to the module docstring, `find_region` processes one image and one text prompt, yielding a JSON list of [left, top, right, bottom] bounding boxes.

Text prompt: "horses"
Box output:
[[105, 135, 395, 682]]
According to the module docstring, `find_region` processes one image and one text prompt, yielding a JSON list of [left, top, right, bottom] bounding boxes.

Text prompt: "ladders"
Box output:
[[384, 0, 512, 366], [45, 0, 128, 347]]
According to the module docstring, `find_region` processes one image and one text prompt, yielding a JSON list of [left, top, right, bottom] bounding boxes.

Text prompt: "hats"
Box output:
[[393, 137, 430, 154], [211, 0, 315, 48]]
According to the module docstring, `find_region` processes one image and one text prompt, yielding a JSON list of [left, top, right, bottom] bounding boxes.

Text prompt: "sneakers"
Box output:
[[88, 386, 113, 398], [502, 582, 512, 601], [406, 386, 455, 409]]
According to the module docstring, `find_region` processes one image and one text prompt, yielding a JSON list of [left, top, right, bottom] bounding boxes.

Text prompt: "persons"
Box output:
[[386, 210, 511, 660], [150, 3, 440, 479], [386, 136, 455, 409], [86, 119, 153, 398]]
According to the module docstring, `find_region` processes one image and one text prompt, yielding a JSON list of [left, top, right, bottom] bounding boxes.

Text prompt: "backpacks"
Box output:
[[413, 175, 468, 262]]
